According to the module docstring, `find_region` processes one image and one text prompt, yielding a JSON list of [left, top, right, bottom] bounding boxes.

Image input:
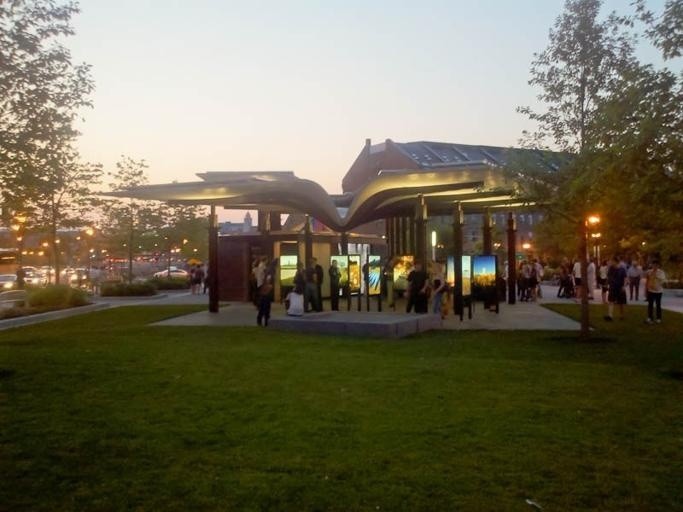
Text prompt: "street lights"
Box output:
[[431, 230, 438, 263], [589, 215, 602, 268]]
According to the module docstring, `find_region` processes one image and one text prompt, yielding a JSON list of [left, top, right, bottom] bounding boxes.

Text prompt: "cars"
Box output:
[[0, 257, 191, 307]]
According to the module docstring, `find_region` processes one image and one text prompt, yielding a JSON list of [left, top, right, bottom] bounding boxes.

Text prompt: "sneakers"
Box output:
[[645, 317, 662, 324]]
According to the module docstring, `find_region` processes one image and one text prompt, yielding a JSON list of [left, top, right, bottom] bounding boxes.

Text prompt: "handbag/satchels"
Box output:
[[595, 275, 607, 285], [434, 279, 451, 293], [518, 277, 529, 289], [440, 302, 449, 319]]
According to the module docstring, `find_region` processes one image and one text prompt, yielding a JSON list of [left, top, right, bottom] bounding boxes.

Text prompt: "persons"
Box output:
[[406, 258, 450, 319], [519, 257, 542, 302], [190, 263, 209, 296], [249, 252, 323, 325], [16, 267, 25, 289], [64, 259, 113, 295], [329, 260, 341, 310], [557, 255, 666, 324]]
